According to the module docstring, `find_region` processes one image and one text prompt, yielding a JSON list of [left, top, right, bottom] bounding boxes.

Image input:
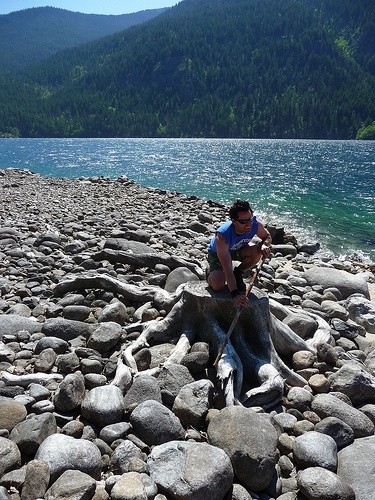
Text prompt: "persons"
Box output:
[[206, 199, 272, 307]]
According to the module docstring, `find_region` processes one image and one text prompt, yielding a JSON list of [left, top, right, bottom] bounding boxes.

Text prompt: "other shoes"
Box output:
[[206, 268, 210, 281], [233, 266, 246, 292]]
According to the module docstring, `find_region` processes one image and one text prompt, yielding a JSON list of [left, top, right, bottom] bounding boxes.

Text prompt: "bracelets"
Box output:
[[230, 289, 241, 299]]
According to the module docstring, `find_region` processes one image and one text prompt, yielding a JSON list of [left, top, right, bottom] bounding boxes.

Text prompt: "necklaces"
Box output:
[[236, 228, 244, 234]]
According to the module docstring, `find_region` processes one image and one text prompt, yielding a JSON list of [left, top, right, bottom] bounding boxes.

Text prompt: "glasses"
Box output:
[[232, 217, 254, 225]]
